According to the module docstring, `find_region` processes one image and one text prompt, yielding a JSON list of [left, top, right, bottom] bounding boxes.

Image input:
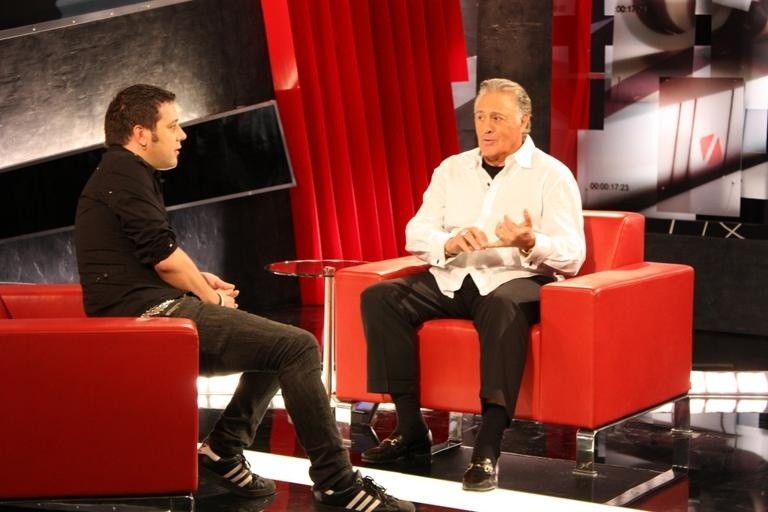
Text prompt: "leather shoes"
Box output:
[[461, 456, 499, 490], [361, 429, 432, 467]]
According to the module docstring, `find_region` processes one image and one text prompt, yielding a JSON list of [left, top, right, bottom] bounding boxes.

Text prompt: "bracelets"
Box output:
[[217, 291, 225, 307], [521, 247, 534, 256]]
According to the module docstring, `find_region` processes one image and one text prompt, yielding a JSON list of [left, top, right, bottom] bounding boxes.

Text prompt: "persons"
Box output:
[[74, 84, 416, 512], [362, 79, 590, 491]]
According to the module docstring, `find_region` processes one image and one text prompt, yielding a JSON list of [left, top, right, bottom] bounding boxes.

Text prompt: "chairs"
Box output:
[[0, 283, 201, 503], [334, 209, 695, 478]]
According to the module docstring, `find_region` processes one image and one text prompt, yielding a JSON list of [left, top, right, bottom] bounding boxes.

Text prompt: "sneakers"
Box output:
[[311, 470, 416, 512], [197, 437, 276, 498]]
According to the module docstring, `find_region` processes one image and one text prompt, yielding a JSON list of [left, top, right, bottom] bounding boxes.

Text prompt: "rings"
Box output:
[[461, 229, 469, 237]]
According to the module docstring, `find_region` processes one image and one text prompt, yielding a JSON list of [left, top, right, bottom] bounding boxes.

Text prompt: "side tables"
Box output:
[[265, 258, 367, 404]]
